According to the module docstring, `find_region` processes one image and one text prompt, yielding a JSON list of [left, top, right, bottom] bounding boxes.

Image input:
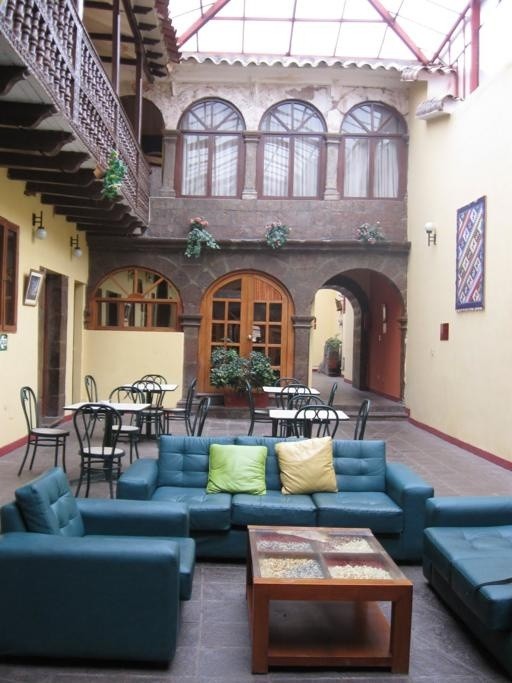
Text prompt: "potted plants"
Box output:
[[91, 146, 127, 201], [209, 348, 277, 398]]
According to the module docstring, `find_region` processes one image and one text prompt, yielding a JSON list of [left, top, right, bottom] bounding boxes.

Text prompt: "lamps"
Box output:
[[32, 210, 47, 241], [70, 233, 82, 258], [424, 221, 436, 246]]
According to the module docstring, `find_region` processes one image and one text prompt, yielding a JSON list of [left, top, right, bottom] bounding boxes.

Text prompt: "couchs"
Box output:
[[112, 438, 440, 568]]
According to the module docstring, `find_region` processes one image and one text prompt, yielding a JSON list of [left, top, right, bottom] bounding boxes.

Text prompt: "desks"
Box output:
[[267, 407, 350, 422], [63, 400, 151, 415], [119, 384, 179, 392], [261, 385, 321, 396]]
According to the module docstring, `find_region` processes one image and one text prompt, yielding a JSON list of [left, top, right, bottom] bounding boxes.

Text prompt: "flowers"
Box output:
[[183, 215, 221, 258], [353, 220, 387, 245], [262, 216, 292, 250]]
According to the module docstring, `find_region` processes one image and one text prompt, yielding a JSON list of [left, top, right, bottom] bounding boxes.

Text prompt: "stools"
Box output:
[[418, 495, 512, 683]]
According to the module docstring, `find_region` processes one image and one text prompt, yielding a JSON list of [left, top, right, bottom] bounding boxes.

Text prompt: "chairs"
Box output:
[[243, 379, 271, 434], [131, 381, 161, 433], [354, 399, 371, 439], [193, 396, 212, 437], [279, 384, 312, 410], [109, 387, 145, 405], [0, 463, 201, 673], [82, 375, 122, 441], [328, 383, 338, 407], [276, 378, 300, 403], [135, 374, 171, 433], [295, 405, 339, 438], [71, 404, 127, 497], [289, 395, 325, 412], [161, 378, 201, 435], [17, 385, 68, 478]]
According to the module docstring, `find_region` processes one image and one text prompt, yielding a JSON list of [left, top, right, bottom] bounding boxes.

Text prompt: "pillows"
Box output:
[[205, 443, 267, 494], [274, 439, 341, 493]]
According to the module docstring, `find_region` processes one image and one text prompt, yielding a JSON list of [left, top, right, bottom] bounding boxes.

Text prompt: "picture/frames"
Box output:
[[123, 303, 131, 322], [23, 268, 46, 306]]
